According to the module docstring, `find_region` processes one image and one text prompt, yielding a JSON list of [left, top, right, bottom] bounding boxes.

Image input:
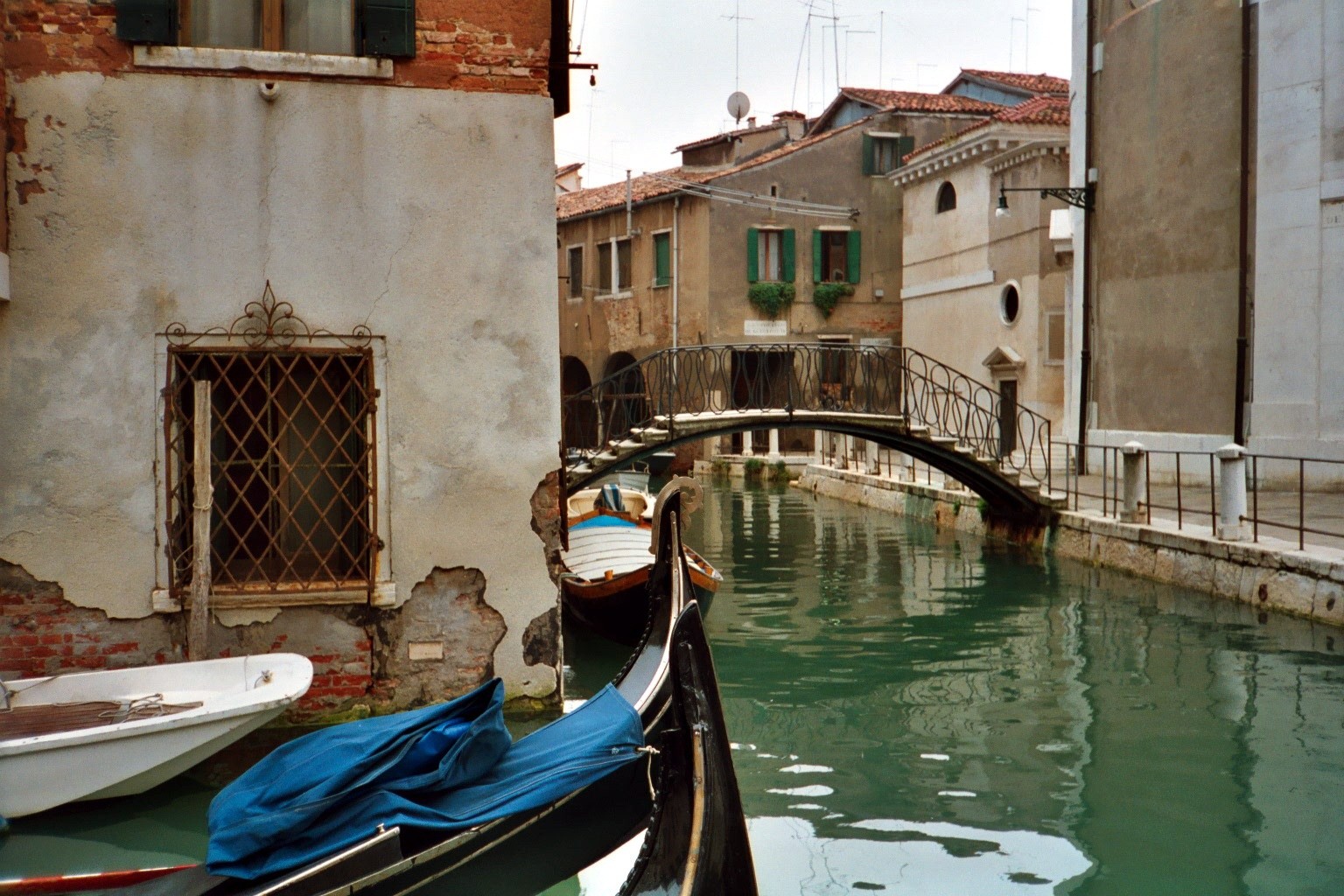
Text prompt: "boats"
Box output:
[[0, 475, 768, 895], [0, 652, 314, 832]]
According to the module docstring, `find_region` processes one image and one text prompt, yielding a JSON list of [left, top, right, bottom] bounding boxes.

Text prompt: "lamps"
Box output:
[[995, 181, 1097, 220]]
